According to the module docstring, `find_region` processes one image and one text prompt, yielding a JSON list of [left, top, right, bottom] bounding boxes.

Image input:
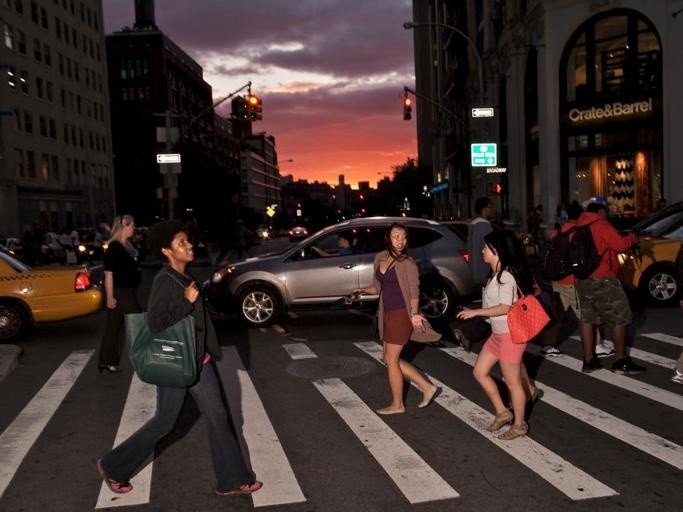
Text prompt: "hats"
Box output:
[[580, 196, 609, 210]]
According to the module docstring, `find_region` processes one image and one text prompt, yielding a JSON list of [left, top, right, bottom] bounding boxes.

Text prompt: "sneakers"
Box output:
[[415, 385, 439, 409], [592, 342, 618, 358], [539, 344, 567, 358], [612, 355, 647, 375], [375, 405, 406, 414], [582, 355, 606, 374], [96, 457, 134, 493], [213, 477, 264, 497]]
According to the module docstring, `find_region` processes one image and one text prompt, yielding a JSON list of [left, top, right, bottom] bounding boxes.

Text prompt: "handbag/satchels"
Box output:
[[504, 293, 551, 345], [123, 270, 201, 388]]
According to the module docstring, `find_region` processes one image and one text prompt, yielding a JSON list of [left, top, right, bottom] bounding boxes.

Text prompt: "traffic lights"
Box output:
[[249, 95, 257, 121], [255, 98, 262, 121], [493, 182, 502, 196], [239, 96, 250, 121], [471, 144, 496, 166], [156, 186, 168, 200], [403, 99, 411, 119]]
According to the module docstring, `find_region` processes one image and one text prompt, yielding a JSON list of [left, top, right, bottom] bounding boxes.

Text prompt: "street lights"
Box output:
[[275, 158, 293, 164], [402, 19, 492, 211]]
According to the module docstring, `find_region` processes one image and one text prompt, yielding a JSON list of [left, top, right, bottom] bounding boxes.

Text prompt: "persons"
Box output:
[[94, 214, 263, 499], [342, 220, 440, 417], [503, 232, 543, 413], [669, 350, 682, 385], [453, 229, 530, 442]]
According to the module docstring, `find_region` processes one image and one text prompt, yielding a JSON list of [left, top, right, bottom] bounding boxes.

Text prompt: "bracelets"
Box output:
[[410, 310, 418, 316]]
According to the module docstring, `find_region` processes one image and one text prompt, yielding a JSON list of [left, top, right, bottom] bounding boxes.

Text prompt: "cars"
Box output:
[[0, 224, 149, 340], [613, 202, 682, 304]]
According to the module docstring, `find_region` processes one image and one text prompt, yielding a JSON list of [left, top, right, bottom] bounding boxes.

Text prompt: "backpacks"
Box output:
[[566, 218, 607, 280], [537, 231, 575, 282]]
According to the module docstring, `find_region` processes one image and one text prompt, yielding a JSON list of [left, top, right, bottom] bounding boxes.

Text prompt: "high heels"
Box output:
[[97, 360, 121, 375], [498, 420, 529, 441], [486, 409, 513, 432]]
